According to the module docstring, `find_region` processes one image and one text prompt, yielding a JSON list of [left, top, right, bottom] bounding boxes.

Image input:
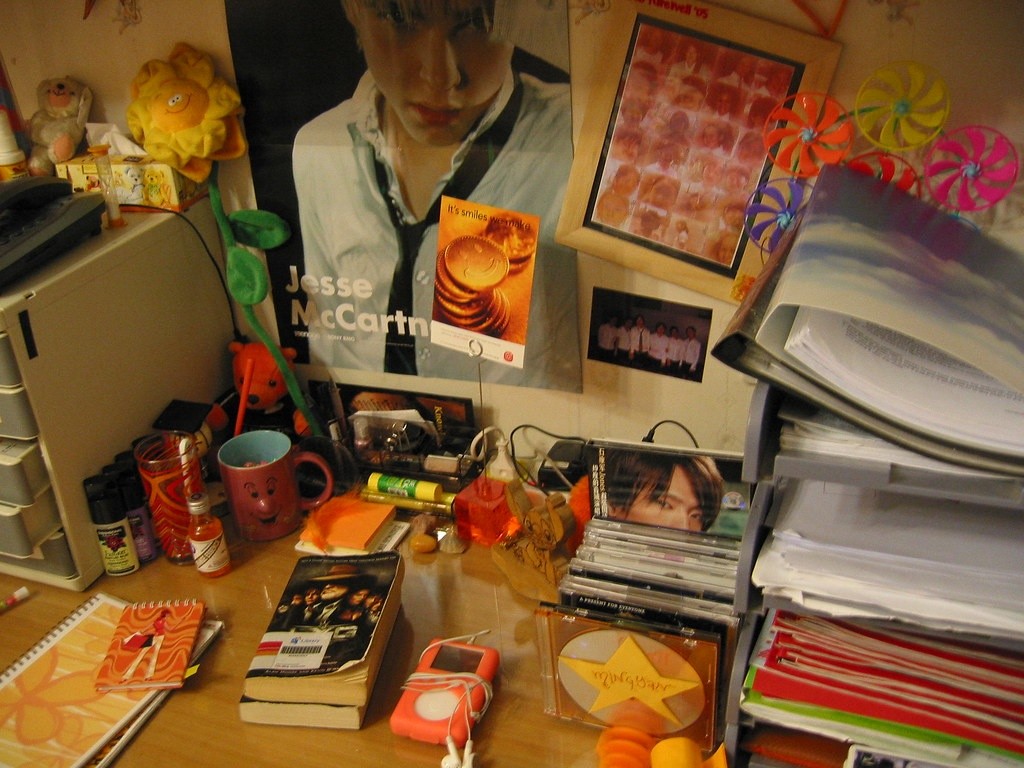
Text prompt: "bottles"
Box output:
[[186, 492, 234, 579], [82, 435, 161, 577]]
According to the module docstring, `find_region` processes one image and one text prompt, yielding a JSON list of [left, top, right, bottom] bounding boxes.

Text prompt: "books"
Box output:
[[740, 613, 1024, 768], [244, 549, 406, 704], [0, 591, 225, 768], [239, 602, 401, 732]]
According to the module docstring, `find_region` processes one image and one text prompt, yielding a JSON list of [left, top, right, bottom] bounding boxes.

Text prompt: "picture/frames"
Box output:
[[553, 0, 842, 303]]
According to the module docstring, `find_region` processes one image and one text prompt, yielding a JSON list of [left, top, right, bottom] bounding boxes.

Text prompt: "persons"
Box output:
[[600, 448, 724, 533], [591, 23, 796, 267], [273, 565, 381, 629], [597, 313, 701, 380], [290, 0, 586, 392]]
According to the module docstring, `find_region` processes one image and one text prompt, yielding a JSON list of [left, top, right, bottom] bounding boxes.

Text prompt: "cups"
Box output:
[[218, 431, 333, 543], [133, 429, 204, 566]]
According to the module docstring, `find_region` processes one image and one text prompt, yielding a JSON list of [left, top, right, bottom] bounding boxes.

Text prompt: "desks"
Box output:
[[0, 497, 727, 768]]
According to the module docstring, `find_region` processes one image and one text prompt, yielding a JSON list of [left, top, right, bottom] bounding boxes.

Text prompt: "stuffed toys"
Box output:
[[25, 75, 92, 176], [210, 342, 313, 445], [126, 41, 322, 436]]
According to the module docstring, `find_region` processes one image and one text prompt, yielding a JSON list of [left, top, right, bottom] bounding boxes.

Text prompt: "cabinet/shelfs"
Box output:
[[0, 198, 234, 589], [721, 367, 1024, 768]]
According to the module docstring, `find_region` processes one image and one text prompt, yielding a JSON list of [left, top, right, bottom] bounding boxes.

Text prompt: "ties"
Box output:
[[374, 68, 523, 375], [637, 328, 644, 354]]
